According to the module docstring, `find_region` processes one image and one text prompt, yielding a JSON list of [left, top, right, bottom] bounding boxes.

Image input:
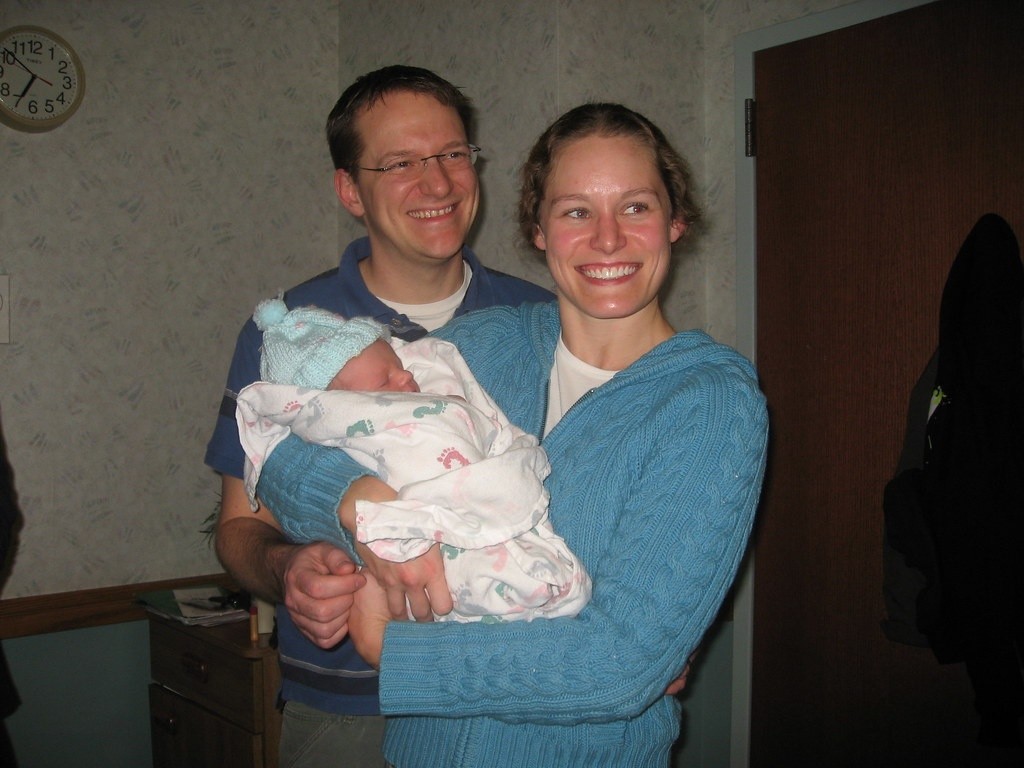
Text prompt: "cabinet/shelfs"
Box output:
[[144, 596, 284, 768]]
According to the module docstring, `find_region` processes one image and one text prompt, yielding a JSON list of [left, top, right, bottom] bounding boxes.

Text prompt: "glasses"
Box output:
[[356, 144, 482, 180]]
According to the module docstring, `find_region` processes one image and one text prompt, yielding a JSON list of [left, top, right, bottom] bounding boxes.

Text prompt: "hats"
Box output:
[[252, 298, 395, 390]]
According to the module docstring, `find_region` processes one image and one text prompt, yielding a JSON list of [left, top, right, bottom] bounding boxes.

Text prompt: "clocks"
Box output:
[[0, 25, 86, 131]]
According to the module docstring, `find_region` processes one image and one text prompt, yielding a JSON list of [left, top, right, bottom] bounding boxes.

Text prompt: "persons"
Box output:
[[253, 299, 592, 622], [205, 65, 703, 768], [244, 102, 769, 768], [0, 425, 24, 768]]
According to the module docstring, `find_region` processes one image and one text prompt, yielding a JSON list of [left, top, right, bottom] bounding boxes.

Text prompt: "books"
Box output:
[[134, 584, 249, 627]]
